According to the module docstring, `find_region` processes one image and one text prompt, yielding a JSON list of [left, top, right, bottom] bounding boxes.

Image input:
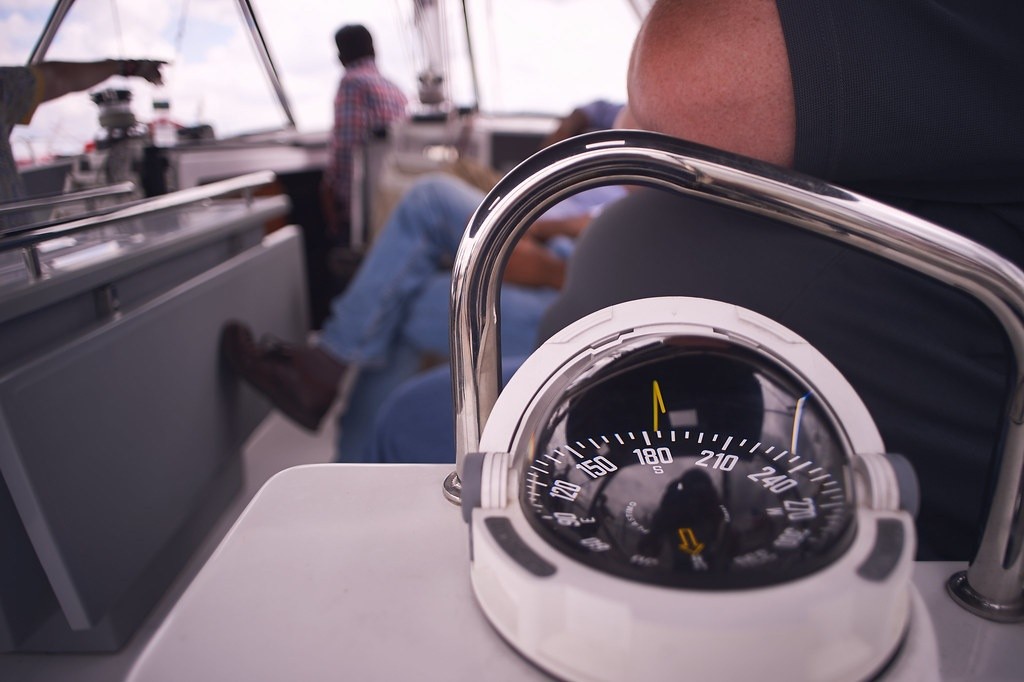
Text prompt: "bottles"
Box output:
[[153, 103, 171, 147]]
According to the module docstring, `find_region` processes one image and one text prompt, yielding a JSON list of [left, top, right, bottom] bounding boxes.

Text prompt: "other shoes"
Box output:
[[221, 320, 351, 433]]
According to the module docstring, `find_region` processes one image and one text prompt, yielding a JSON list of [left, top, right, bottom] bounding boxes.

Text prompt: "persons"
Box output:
[[224, 1, 1024, 562], [0, 58, 169, 200]]
